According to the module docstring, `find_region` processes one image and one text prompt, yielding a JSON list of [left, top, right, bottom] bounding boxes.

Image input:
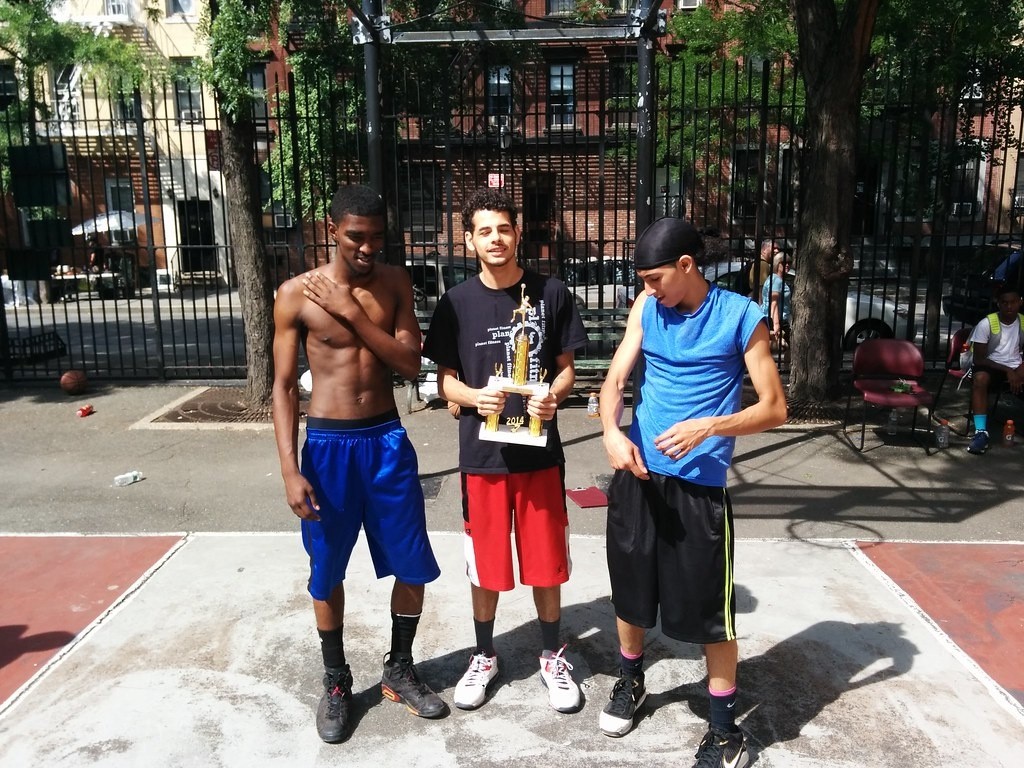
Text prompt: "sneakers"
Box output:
[[316, 664, 353, 743], [965, 430, 990, 454], [691, 722, 750, 768], [380, 652, 447, 718], [598, 668, 647, 737], [453, 651, 500, 709], [538, 643, 581, 713]]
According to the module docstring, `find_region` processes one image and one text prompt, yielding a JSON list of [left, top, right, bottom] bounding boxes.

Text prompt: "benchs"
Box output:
[[397, 307, 643, 413]]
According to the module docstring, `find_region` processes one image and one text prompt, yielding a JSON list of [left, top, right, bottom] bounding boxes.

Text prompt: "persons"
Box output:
[[749, 239, 779, 303], [966, 288, 1024, 454], [760, 252, 791, 362], [272, 186, 445, 744], [87, 235, 104, 273], [419, 190, 593, 714], [600, 218, 788, 768]]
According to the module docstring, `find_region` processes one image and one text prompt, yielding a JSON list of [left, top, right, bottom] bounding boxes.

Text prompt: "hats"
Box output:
[[634, 216, 704, 270]]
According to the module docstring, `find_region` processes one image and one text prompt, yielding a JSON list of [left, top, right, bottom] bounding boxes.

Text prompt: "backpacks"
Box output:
[[960, 312, 1024, 372], [733, 259, 765, 296]]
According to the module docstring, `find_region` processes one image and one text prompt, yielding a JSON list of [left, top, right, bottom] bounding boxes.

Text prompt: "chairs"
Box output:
[[933, 327, 1001, 436], [843, 339, 935, 456]]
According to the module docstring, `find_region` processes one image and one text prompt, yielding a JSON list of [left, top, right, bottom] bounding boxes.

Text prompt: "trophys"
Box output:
[[479, 284, 550, 448]]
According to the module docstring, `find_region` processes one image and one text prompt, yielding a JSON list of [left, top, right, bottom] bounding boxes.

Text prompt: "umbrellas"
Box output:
[[71, 210, 160, 236]]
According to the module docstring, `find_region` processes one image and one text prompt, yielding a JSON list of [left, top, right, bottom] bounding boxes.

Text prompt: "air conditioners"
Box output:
[[182, 109, 202, 124], [677, 0, 703, 9], [492, 115, 509, 127], [952, 202, 973, 216], [274, 213, 292, 228]]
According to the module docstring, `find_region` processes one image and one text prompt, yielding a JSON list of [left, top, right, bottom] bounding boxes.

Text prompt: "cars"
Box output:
[[404, 252, 483, 336], [696, 233, 919, 357], [940, 240, 1024, 329], [517, 255, 642, 334]]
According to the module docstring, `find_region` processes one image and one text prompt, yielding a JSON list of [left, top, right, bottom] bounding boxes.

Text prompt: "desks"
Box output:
[[51, 273, 122, 302]]
